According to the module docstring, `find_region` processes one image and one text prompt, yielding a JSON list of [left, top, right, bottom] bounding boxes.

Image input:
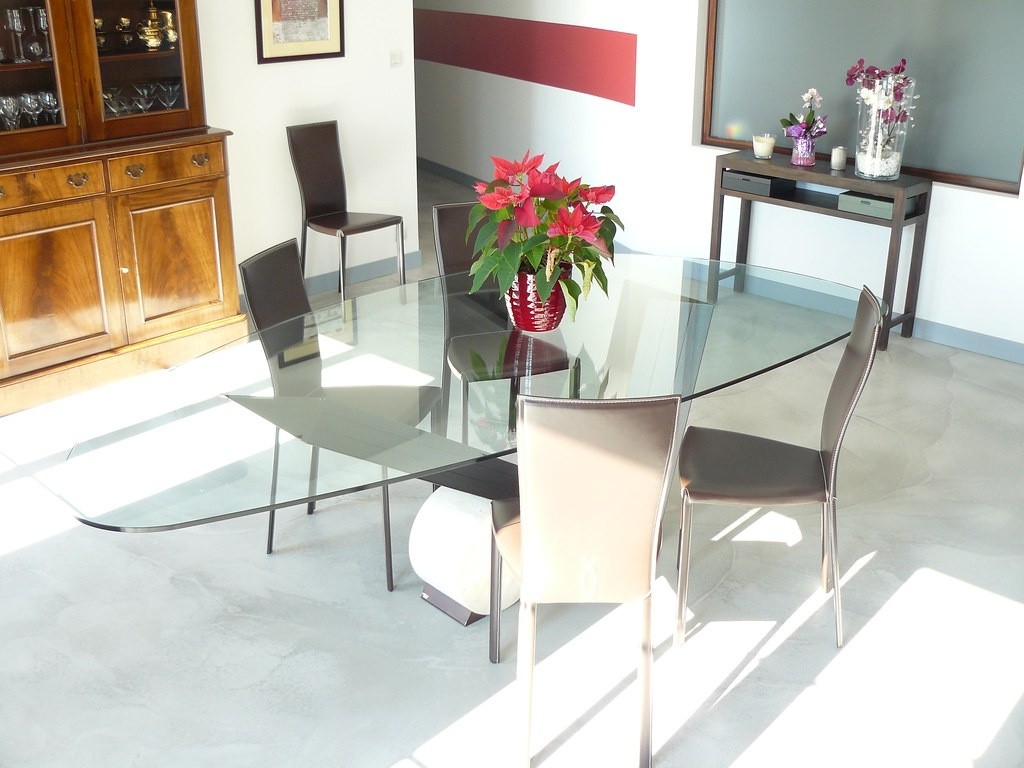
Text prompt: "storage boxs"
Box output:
[[837, 190, 919, 221], [721, 170, 797, 196]]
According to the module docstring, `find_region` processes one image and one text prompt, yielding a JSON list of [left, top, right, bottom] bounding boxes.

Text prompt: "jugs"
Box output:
[[135, 0, 179, 51]]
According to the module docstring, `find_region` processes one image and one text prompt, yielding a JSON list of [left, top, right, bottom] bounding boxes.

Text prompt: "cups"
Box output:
[[752, 133, 777, 160], [830, 144, 848, 171], [94, 17, 138, 54]]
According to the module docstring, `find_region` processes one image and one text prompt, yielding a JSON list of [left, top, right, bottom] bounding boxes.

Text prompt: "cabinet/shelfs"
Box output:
[[0, 0, 211, 165], [0, 126, 249, 418]]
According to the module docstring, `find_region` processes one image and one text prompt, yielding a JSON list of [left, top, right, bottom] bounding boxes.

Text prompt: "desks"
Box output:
[[706, 147, 935, 351], [54, 256, 889, 629]]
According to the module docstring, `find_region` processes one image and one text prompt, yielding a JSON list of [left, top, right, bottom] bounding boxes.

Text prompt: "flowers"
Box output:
[[846, 59, 913, 152], [780, 88, 828, 155], [465, 147, 625, 323]]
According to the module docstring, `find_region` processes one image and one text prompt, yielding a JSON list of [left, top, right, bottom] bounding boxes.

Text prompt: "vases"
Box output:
[[504, 262, 575, 332], [855, 76, 914, 181], [789, 137, 816, 166]]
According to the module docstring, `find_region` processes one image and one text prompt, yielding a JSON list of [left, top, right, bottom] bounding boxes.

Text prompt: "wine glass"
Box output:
[[102, 79, 182, 117], [0, 88, 60, 129], [3, 6, 52, 64]]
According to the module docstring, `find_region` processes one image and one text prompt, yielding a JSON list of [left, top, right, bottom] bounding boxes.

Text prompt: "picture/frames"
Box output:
[[254, 0, 345, 64]]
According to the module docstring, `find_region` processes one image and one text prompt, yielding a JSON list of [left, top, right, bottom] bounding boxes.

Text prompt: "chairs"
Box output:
[[674, 285, 884, 651], [285, 119, 407, 323], [488, 381, 682, 768], [428, 202, 581, 445], [238, 238, 443, 592]]
